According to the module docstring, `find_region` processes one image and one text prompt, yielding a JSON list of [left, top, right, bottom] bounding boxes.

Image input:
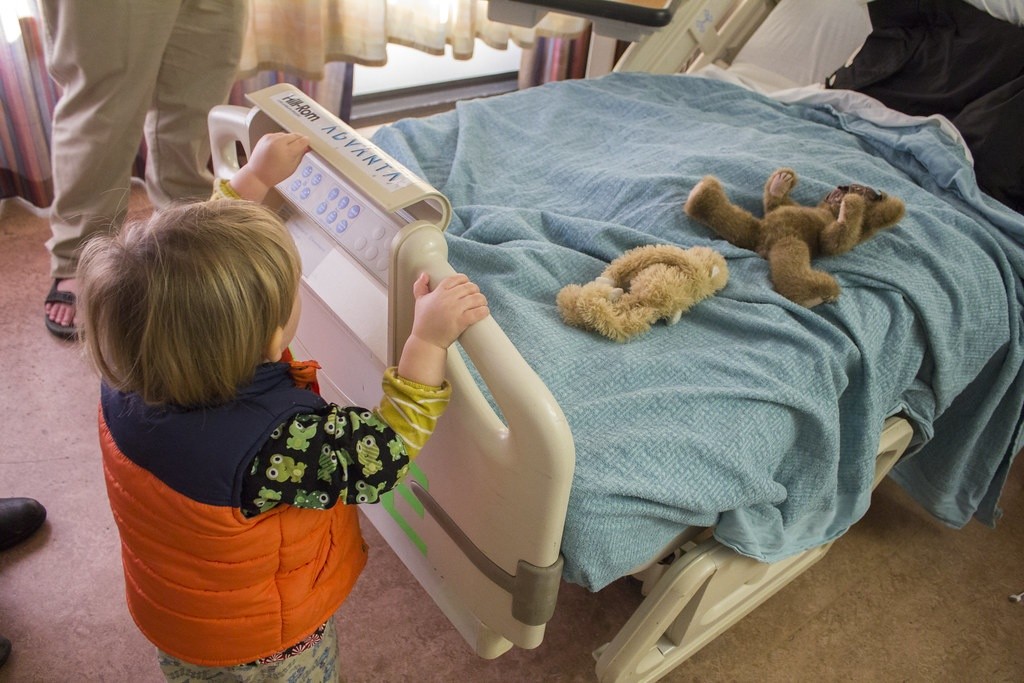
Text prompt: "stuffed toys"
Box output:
[[683, 167, 905, 309], [556, 244, 729, 344]]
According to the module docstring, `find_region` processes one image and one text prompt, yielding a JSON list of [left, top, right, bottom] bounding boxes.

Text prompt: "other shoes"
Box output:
[[0, 497, 46, 553]]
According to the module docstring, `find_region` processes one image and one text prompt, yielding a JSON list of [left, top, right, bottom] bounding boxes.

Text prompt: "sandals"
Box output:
[[44, 278, 85, 337]]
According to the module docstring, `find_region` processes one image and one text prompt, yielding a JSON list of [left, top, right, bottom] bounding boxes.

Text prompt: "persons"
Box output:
[[75, 132, 490, 683], [37, 0, 249, 338], [0, 495, 46, 672]]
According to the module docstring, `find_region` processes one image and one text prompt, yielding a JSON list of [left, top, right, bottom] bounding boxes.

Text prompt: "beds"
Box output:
[[208, 1, 1024, 683]]
